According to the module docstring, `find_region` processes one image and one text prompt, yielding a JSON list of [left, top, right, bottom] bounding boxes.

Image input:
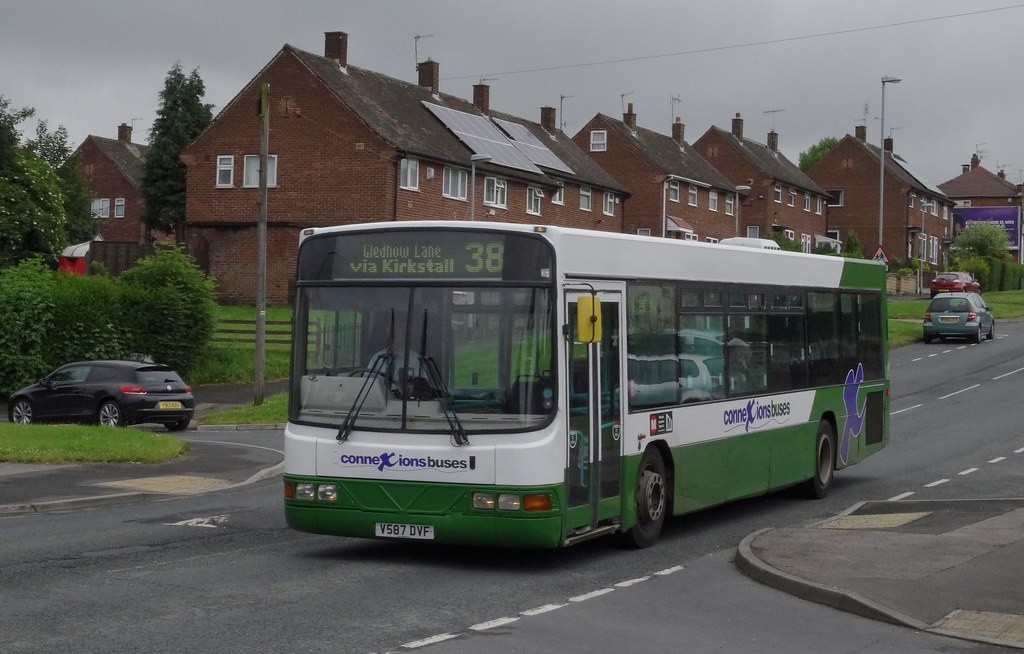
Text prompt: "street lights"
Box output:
[[918, 203, 933, 295], [878, 75, 903, 247], [734, 183, 752, 230], [469, 152, 492, 222]]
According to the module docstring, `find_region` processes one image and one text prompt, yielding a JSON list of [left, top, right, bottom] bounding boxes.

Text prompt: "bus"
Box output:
[[282, 219, 893, 550]]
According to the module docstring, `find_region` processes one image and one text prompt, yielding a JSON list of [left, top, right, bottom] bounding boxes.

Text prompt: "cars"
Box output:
[[929, 272, 982, 299], [6, 359, 195, 432], [923, 292, 996, 344]]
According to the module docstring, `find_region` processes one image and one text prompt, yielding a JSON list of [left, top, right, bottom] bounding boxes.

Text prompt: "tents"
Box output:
[[58, 234, 105, 275]]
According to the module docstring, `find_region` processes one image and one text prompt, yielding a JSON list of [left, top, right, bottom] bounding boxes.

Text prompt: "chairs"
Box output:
[[508, 370, 552, 417]]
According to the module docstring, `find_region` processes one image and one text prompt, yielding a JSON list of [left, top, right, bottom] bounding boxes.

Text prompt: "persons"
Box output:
[[731, 294, 767, 341], [733, 343, 766, 392], [369, 284, 443, 357], [774, 338, 792, 391], [363, 320, 433, 400], [938, 298, 954, 312]]
